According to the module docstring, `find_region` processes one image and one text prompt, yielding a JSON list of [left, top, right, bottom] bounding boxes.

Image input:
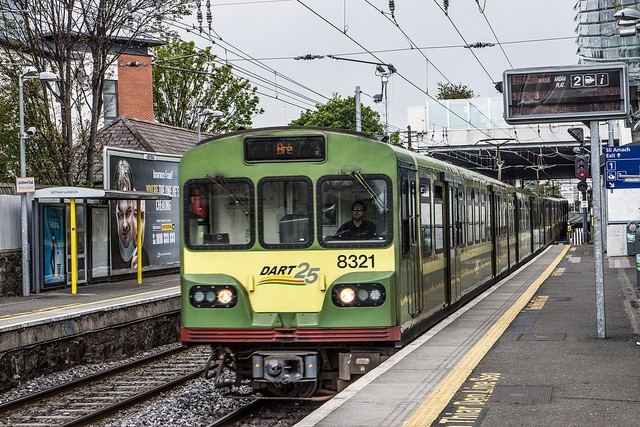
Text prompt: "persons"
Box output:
[[131, 200, 149, 267], [335, 200, 376, 240], [47, 218, 59, 277], [112, 159, 138, 269]]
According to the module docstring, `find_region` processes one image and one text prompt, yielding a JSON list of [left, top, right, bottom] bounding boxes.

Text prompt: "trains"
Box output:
[[179, 127, 570, 401]]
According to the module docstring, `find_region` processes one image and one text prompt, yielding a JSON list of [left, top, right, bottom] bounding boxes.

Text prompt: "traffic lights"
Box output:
[[576, 157, 587, 192]]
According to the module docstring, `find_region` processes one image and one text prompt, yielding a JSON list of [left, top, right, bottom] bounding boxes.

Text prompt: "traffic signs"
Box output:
[[502, 62, 630, 124], [606, 146, 640, 189]]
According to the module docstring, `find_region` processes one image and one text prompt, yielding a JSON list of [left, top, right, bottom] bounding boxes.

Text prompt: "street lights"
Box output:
[[19, 71, 56, 297], [614, 8, 639, 20], [567, 128, 589, 243]]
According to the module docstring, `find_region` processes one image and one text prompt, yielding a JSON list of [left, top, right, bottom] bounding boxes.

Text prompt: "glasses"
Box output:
[[353, 208, 363, 211]]
